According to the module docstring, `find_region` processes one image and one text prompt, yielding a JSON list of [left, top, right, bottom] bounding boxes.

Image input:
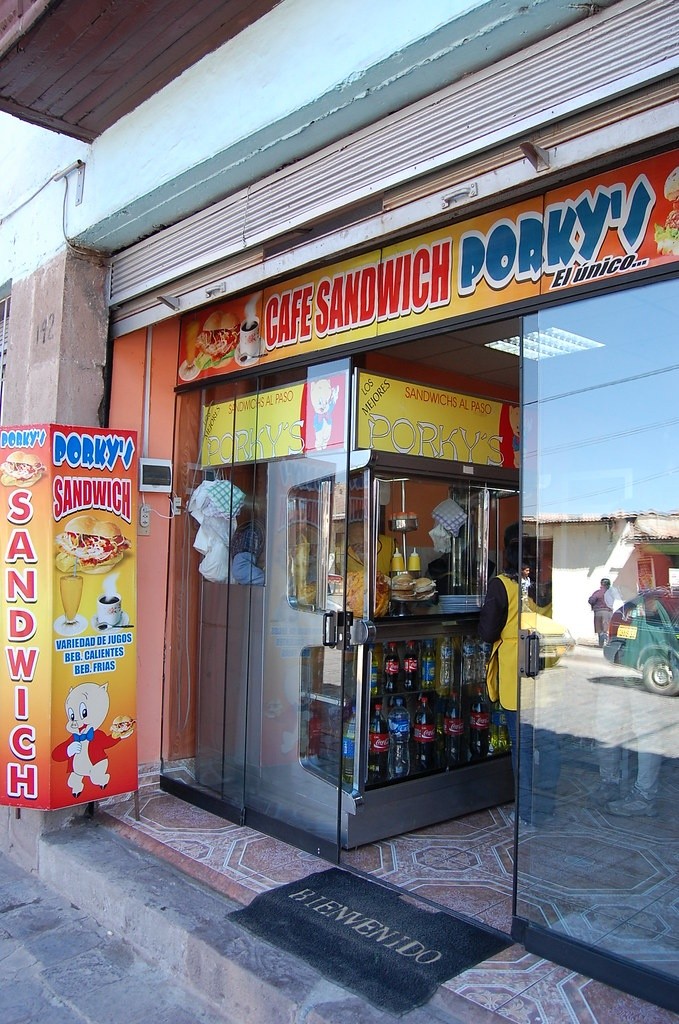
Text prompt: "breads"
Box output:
[[298, 568, 389, 618]]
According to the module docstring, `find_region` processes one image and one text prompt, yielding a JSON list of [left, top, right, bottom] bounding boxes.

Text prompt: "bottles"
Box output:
[[341, 707, 356, 785], [412, 697, 435, 772], [345, 633, 492, 695], [386, 698, 411, 780], [408, 547, 421, 571], [436, 688, 511, 759], [391, 548, 404, 571], [368, 705, 390, 783]]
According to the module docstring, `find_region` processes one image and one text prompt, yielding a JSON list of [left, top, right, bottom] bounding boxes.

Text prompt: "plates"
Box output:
[[53, 613, 88, 636], [235, 336, 265, 367], [92, 610, 129, 635]]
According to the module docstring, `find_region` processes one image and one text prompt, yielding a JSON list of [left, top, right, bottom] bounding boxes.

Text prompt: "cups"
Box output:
[[239, 316, 260, 359], [60, 575, 83, 633], [97, 594, 123, 625]]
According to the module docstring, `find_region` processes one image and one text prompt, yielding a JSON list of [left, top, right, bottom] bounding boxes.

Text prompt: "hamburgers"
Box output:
[[56, 515, 132, 575], [191, 309, 241, 369], [391, 574, 437, 592], [1, 451, 46, 488], [652, 165, 679, 256], [110, 715, 137, 739]]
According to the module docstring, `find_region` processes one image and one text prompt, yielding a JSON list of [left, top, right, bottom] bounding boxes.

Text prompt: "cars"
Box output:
[[603, 586, 679, 696], [521, 603, 576, 667]]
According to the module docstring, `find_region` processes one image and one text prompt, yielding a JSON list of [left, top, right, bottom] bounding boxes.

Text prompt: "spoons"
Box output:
[[239, 353, 269, 362], [98, 622, 134, 630]]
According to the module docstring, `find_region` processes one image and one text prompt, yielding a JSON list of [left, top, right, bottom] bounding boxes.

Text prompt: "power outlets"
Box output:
[[137, 504, 151, 535]]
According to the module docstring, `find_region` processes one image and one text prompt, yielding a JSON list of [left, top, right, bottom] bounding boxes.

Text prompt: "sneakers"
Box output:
[[587, 780, 620, 805], [604, 781, 658, 817]]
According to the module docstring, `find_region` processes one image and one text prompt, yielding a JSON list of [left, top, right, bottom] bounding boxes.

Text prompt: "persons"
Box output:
[[587, 578, 616, 647], [586, 545, 679, 817], [513, 563, 532, 614], [473, 523, 558, 828]]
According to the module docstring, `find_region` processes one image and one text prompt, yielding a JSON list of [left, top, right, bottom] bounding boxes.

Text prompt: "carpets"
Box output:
[[225, 867, 515, 1019]]
